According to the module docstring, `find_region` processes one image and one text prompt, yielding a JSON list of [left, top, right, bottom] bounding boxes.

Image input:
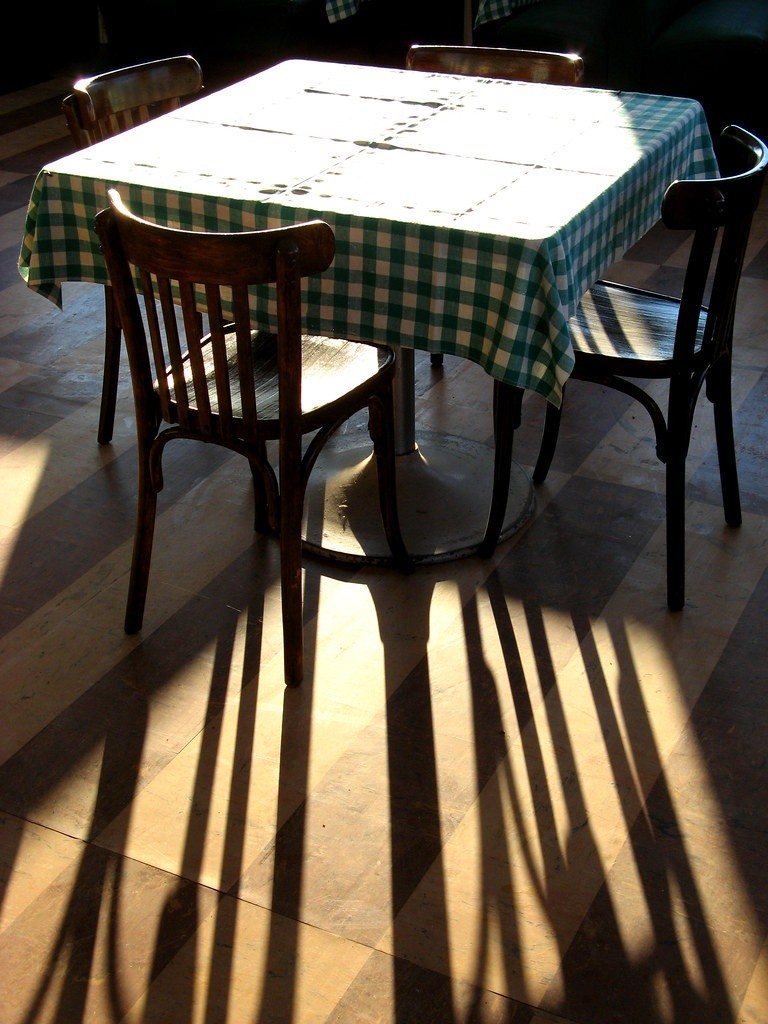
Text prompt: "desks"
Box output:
[[18, 58, 722, 574]]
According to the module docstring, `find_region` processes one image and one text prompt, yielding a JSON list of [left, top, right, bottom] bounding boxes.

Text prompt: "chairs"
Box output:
[[475, 124, 768, 615], [93, 188, 412, 686], [408, 44, 586, 365], [62, 56, 208, 444]]
